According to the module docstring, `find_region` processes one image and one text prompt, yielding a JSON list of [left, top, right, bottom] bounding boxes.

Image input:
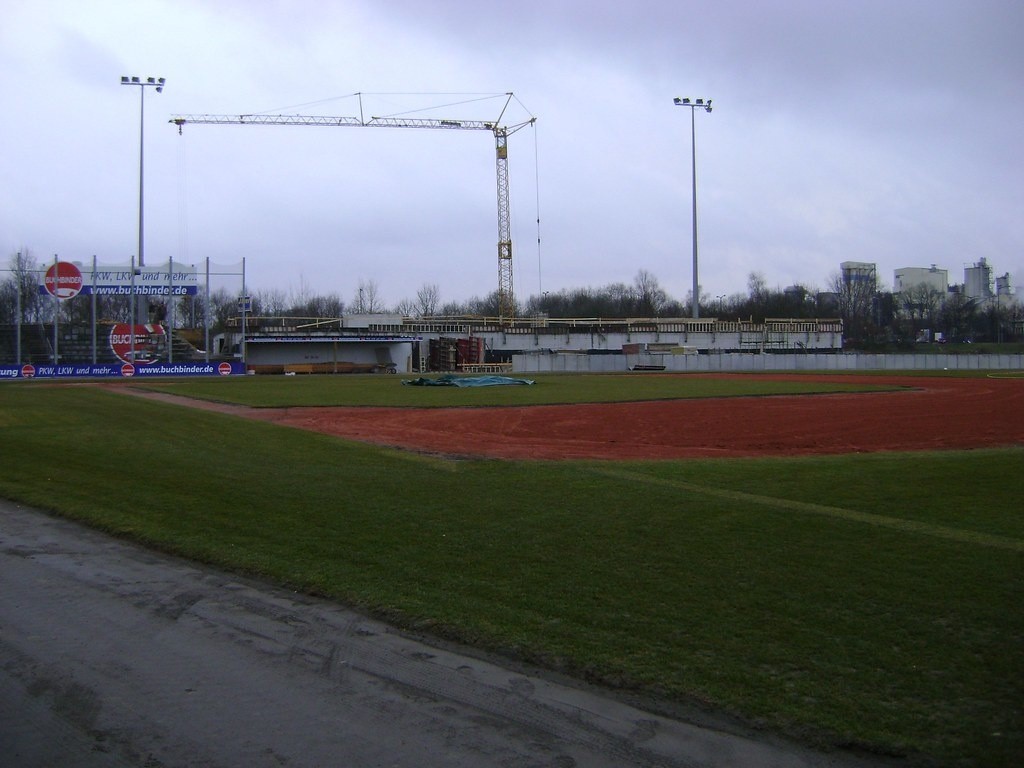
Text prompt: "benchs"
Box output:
[[629, 365, 666, 371], [0, 320, 195, 367]]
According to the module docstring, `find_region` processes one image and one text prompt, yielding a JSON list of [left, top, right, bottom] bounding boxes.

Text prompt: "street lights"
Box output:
[[118, 76, 167, 271], [672, 98, 713, 319]]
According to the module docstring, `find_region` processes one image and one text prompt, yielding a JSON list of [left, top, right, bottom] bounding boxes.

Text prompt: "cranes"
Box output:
[[167, 90, 540, 325]]
